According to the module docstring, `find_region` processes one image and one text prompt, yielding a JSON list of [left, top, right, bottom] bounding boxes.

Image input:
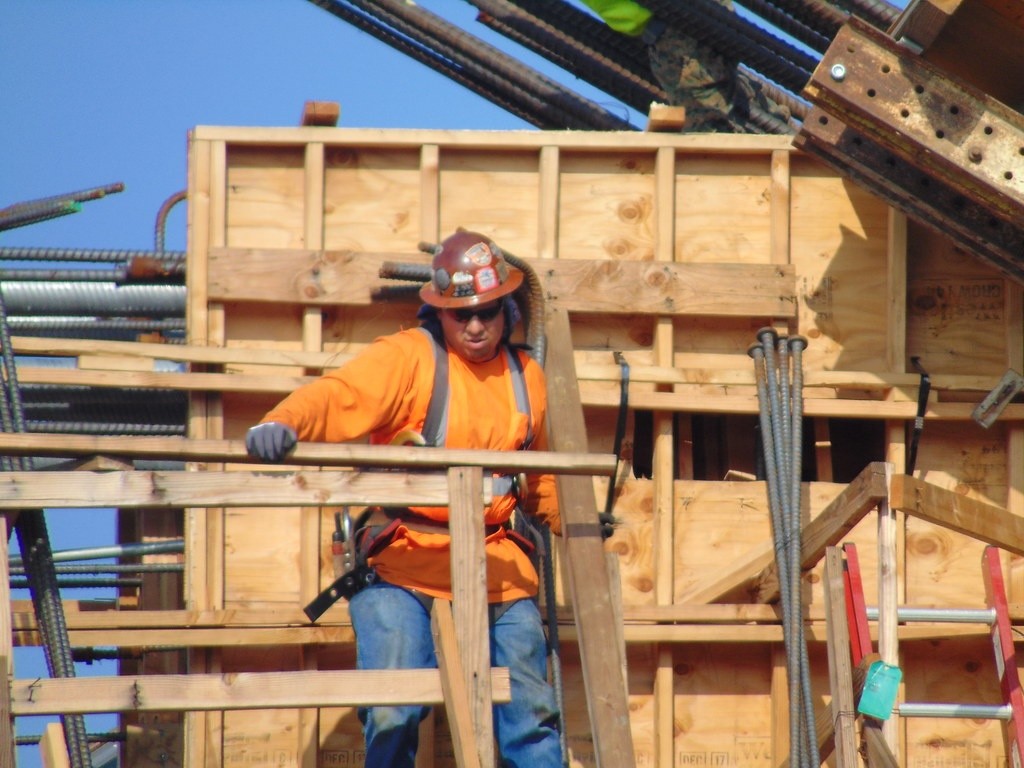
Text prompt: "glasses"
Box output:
[[446, 301, 503, 323]]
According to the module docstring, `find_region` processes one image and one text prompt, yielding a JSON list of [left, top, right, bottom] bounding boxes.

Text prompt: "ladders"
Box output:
[[816, 538, 1024, 768]]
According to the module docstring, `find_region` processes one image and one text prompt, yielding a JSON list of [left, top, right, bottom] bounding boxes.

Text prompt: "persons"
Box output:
[[586, 0, 750, 136], [240, 222, 615, 768]]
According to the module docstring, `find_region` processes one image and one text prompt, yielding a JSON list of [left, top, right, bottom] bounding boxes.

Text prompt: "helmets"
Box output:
[[419, 229, 524, 309]]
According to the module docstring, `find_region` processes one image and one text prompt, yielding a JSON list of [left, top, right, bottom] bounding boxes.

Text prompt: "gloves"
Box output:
[[598, 512, 615, 543], [245, 423, 297, 462]]
